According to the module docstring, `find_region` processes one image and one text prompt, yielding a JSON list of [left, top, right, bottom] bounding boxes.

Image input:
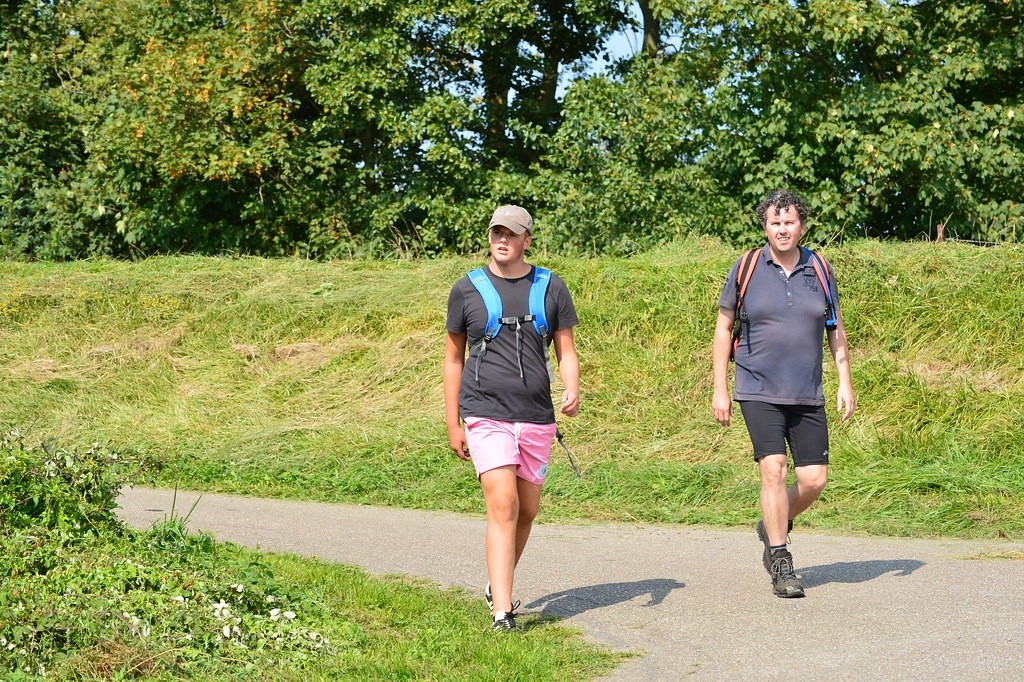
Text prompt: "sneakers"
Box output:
[[756, 520, 804, 598], [485, 581, 520, 636]]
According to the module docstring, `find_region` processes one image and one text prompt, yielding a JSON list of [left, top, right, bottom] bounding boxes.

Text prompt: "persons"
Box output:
[[712, 190, 855, 598], [444, 205, 580, 634]]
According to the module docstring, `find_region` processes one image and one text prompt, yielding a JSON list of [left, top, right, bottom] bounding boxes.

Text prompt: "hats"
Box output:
[[487, 206, 534, 237]]
